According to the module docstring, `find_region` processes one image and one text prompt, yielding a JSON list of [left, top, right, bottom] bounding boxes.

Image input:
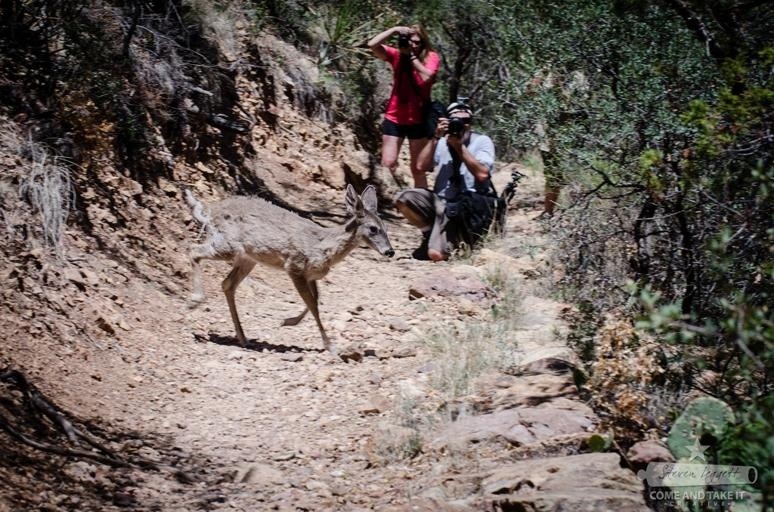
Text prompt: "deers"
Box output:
[[183, 181, 395, 351]]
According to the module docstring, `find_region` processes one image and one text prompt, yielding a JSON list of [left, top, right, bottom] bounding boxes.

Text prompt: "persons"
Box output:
[[368, 25, 440, 189], [396, 102, 496, 262], [525, 45, 591, 220]]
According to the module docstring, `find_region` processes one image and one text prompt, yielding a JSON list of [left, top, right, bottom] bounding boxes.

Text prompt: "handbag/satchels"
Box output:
[[420, 98, 449, 140], [457, 190, 506, 249]]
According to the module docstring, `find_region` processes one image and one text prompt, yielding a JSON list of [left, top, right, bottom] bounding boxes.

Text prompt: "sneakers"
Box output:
[[532, 211, 553, 221], [419, 236, 432, 253]]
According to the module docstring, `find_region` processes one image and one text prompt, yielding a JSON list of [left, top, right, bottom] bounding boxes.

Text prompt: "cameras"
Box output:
[[448, 118, 466, 138], [399, 34, 410, 49]]
